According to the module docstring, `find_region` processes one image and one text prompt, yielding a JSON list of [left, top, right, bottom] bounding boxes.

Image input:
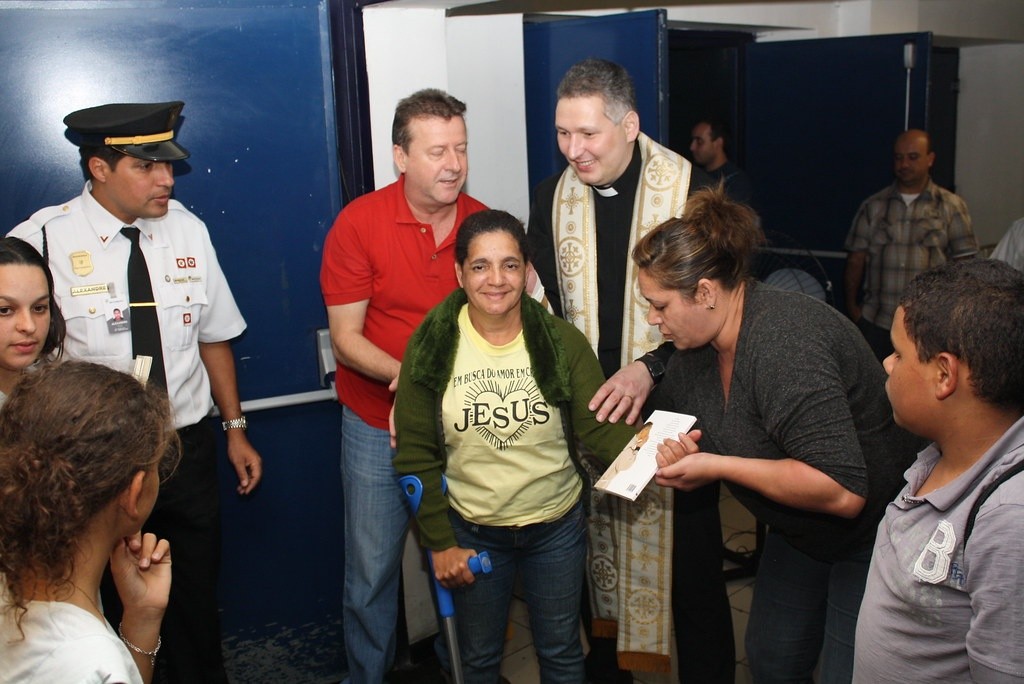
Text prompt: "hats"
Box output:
[[63, 101, 191, 162]]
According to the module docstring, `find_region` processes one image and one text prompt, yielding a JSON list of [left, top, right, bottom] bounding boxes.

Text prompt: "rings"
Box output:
[[621, 395, 633, 402]]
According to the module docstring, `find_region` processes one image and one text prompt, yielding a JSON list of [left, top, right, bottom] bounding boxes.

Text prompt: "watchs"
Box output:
[[222, 416, 248, 431], [634, 354, 666, 387]]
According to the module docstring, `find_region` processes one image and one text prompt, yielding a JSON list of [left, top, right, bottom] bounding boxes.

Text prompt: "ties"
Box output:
[[120, 227, 170, 415]]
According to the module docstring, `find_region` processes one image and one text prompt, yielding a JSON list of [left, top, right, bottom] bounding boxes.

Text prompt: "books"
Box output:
[[593, 409, 697, 501], [132, 355, 152, 390]]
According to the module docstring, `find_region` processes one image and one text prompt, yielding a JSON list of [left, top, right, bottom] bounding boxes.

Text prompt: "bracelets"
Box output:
[[119, 623, 161, 667]]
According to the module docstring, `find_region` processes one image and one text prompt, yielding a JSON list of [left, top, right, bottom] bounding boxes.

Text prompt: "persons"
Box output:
[[392, 209, 700, 684], [113, 308, 123, 321], [0, 100, 263, 684], [594, 421, 654, 489], [633, 182, 918, 684], [320, 89, 489, 684], [0, 236, 67, 405], [843, 130, 1024, 365], [851, 261, 1024, 684], [526, 63, 706, 684], [689, 121, 757, 212], [0, 362, 171, 684]]
